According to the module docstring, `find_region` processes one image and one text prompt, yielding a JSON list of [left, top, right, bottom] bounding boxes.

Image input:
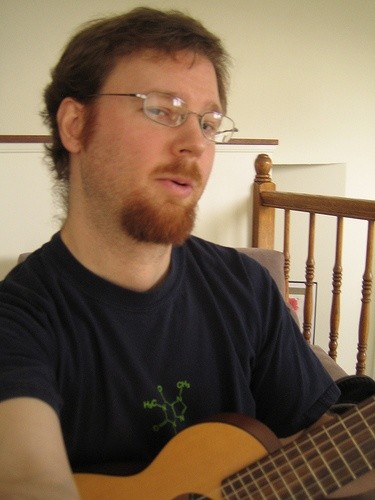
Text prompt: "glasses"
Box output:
[[85, 88, 239, 145]]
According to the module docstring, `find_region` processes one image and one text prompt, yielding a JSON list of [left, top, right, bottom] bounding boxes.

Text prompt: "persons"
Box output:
[[0, 9, 374, 500]]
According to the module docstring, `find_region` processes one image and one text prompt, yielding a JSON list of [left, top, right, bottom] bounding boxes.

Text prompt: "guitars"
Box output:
[[72, 393, 375, 500]]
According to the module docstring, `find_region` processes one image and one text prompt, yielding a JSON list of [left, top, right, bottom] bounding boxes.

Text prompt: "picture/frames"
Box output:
[[288, 280, 317, 345]]
